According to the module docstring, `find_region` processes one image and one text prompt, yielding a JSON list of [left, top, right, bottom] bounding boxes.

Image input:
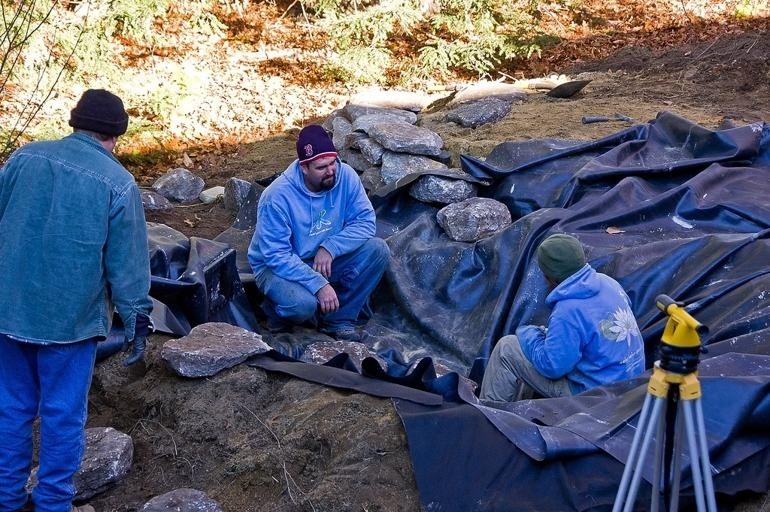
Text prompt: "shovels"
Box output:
[[527, 80, 590, 97]]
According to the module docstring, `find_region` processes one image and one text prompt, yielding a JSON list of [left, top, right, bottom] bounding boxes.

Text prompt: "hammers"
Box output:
[[583, 111, 633, 125]]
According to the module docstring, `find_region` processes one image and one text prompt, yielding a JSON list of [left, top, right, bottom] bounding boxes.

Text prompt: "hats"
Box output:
[[68, 89, 129, 137], [537, 233, 585, 283], [296, 125, 338, 165]]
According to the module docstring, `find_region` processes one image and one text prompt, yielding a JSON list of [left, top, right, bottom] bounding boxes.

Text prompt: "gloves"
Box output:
[[120, 333, 146, 368]]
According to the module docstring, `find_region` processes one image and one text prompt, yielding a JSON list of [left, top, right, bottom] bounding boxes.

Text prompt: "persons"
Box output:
[[479, 231, 647, 409], [1, 87, 158, 511], [246, 125, 391, 339]]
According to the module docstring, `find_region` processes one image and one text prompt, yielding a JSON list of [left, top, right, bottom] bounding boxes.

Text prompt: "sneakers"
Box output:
[[336, 327, 361, 344], [73, 504, 96, 512]]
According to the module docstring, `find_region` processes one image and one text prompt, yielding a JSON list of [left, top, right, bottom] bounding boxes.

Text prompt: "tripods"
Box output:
[[612, 359, 718, 512]]
